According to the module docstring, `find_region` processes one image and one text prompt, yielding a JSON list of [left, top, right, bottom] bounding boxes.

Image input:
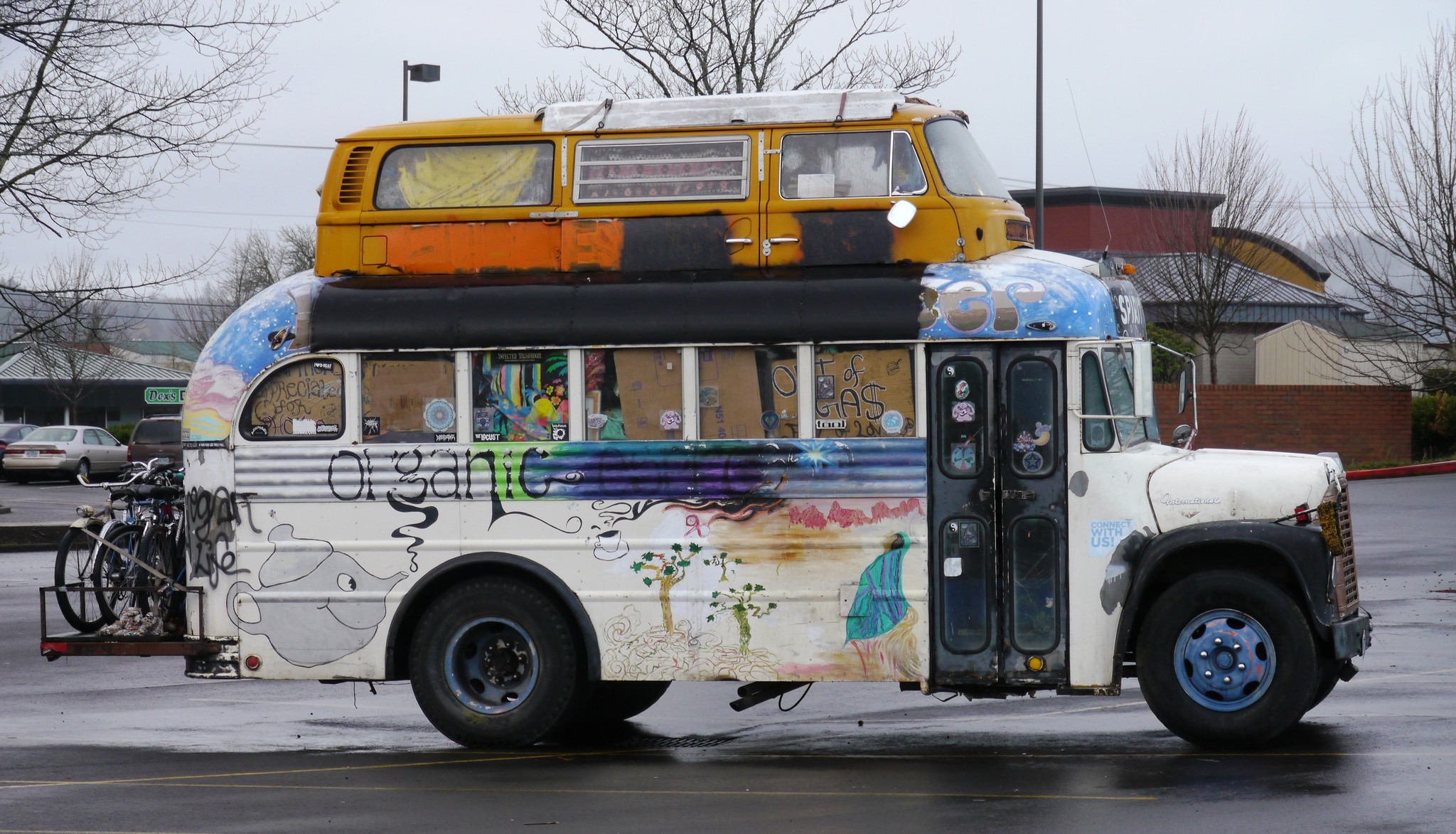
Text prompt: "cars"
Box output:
[[2, 425, 129, 483], [0, 424, 42, 473]]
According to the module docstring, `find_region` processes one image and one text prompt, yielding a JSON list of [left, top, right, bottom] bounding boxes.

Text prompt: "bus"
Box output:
[[174, 86, 1367, 756]]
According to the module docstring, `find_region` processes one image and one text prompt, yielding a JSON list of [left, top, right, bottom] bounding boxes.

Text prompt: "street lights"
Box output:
[[404, 60, 440, 121]]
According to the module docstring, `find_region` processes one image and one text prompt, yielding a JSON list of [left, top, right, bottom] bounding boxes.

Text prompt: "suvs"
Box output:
[[128, 414, 182, 470]]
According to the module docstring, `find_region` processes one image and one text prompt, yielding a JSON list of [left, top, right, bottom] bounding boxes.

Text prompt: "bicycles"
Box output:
[[58, 454, 182, 640]]
